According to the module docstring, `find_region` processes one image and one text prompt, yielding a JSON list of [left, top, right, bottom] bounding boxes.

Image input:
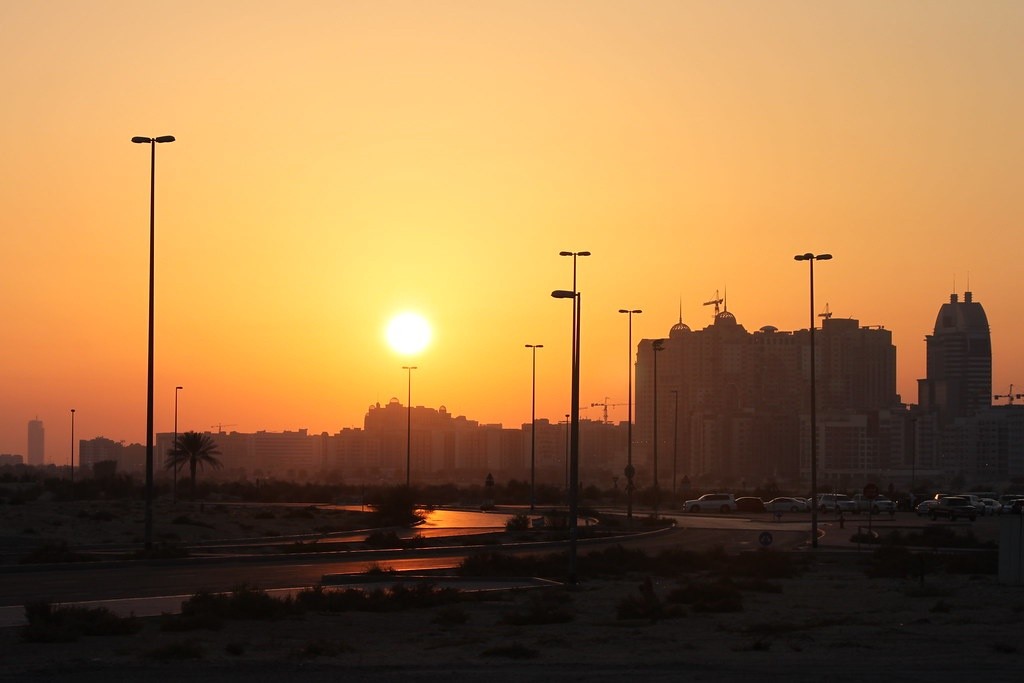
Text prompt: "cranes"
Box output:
[[993, 383, 1024, 406]]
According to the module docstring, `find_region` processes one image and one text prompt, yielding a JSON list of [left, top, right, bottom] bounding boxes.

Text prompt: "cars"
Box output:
[[799, 489, 1024, 523], [763, 496, 806, 513], [734, 496, 765, 513]]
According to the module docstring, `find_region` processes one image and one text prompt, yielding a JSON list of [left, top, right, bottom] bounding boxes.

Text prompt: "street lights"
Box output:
[[70, 408, 75, 483], [793, 252, 833, 550], [402, 366, 417, 484], [173, 386, 182, 504], [129, 135, 176, 549], [671, 388, 679, 498], [550, 288, 581, 565], [559, 250, 591, 371], [524, 343, 543, 514], [564, 413, 569, 489], [650, 337, 665, 520], [618, 309, 643, 521]]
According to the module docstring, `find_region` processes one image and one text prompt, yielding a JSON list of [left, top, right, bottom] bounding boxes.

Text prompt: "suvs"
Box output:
[[682, 492, 736, 515]]
